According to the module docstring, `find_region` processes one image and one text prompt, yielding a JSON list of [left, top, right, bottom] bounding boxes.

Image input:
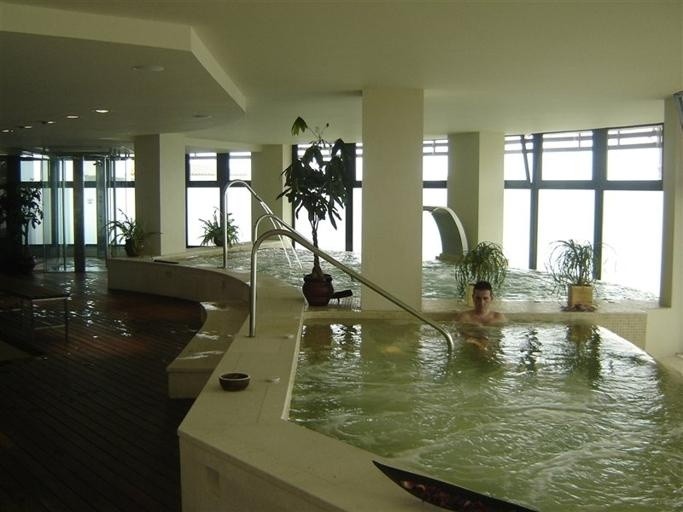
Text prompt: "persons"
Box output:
[[452, 280, 507, 325]]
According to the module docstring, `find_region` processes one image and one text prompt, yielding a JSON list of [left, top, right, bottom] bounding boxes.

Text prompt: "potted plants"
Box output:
[[100, 207, 164, 258], [272, 114, 357, 311], [454, 239, 509, 310], [0, 178, 48, 271], [197, 208, 241, 247], [541, 238, 620, 308]]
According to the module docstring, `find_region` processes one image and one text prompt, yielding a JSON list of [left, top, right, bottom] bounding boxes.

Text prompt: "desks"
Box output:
[[0, 276, 73, 353]]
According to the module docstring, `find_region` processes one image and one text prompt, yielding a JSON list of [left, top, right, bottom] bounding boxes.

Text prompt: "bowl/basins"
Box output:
[[218, 372, 251, 393]]
